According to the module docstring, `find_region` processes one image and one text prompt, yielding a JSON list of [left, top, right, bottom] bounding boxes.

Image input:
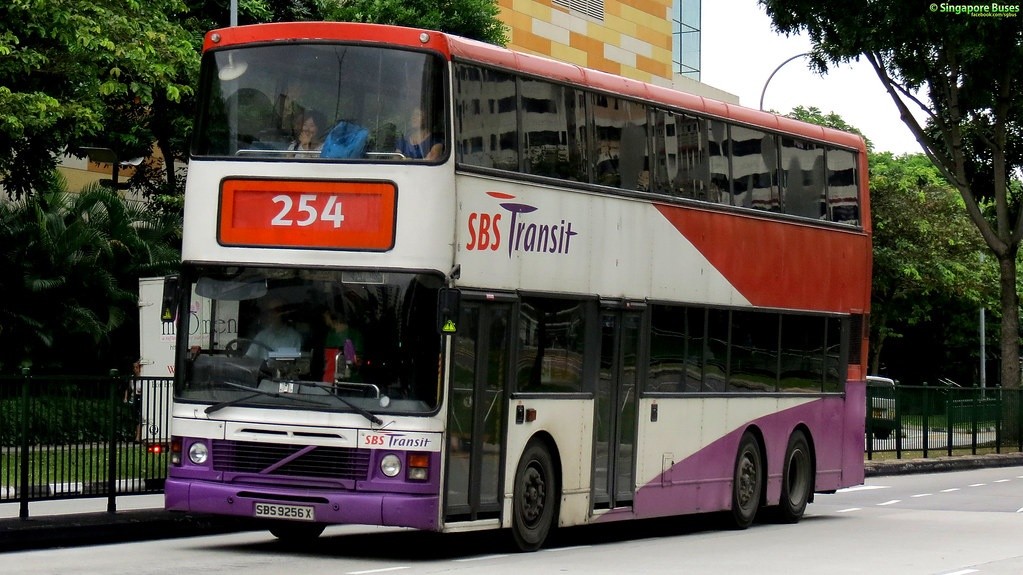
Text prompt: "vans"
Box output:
[[864, 375, 896, 440]]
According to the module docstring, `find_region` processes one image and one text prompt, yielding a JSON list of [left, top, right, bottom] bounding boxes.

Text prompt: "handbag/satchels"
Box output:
[[320, 119, 371, 161]]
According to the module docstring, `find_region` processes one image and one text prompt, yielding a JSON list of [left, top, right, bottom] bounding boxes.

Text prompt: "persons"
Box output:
[[245, 299, 303, 366], [324, 301, 364, 381], [124, 361, 141, 443], [286, 110, 326, 157], [393, 104, 443, 160]]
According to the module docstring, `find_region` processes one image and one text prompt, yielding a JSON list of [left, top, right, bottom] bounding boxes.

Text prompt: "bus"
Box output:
[[159, 19, 873, 551]]
